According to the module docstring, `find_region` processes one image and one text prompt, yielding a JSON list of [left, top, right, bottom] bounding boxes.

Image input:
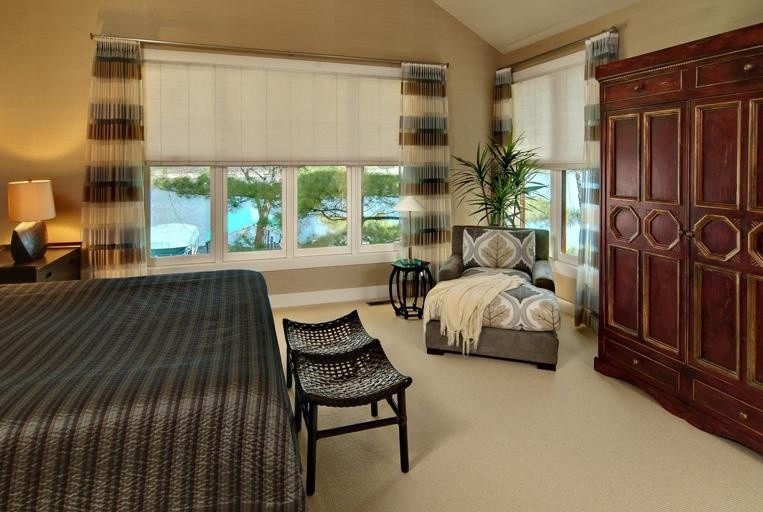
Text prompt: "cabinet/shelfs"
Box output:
[[592, 21, 763, 456]]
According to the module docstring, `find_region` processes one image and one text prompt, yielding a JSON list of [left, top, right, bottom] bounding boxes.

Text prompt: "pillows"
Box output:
[[461, 226, 538, 272]]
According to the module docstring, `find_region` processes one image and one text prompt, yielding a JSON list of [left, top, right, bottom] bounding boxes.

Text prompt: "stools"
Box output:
[[422, 279, 562, 370]]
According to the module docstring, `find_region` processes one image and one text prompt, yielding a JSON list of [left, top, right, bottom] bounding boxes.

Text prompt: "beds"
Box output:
[[1, 269, 306, 509]]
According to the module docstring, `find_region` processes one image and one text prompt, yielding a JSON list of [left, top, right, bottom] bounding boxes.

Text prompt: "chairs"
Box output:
[[422, 224, 561, 291]]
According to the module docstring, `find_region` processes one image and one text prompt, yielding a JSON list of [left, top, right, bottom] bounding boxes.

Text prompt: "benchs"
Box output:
[[283, 306, 412, 494]]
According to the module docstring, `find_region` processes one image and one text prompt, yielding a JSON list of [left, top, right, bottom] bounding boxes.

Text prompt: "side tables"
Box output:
[[389, 258, 435, 319]]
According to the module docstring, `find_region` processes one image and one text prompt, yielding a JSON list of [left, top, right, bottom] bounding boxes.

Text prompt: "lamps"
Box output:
[[7, 178, 56, 261], [393, 196, 426, 264]]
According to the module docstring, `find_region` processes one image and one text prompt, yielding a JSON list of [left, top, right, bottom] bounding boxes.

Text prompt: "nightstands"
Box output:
[[0, 247, 81, 281]]
